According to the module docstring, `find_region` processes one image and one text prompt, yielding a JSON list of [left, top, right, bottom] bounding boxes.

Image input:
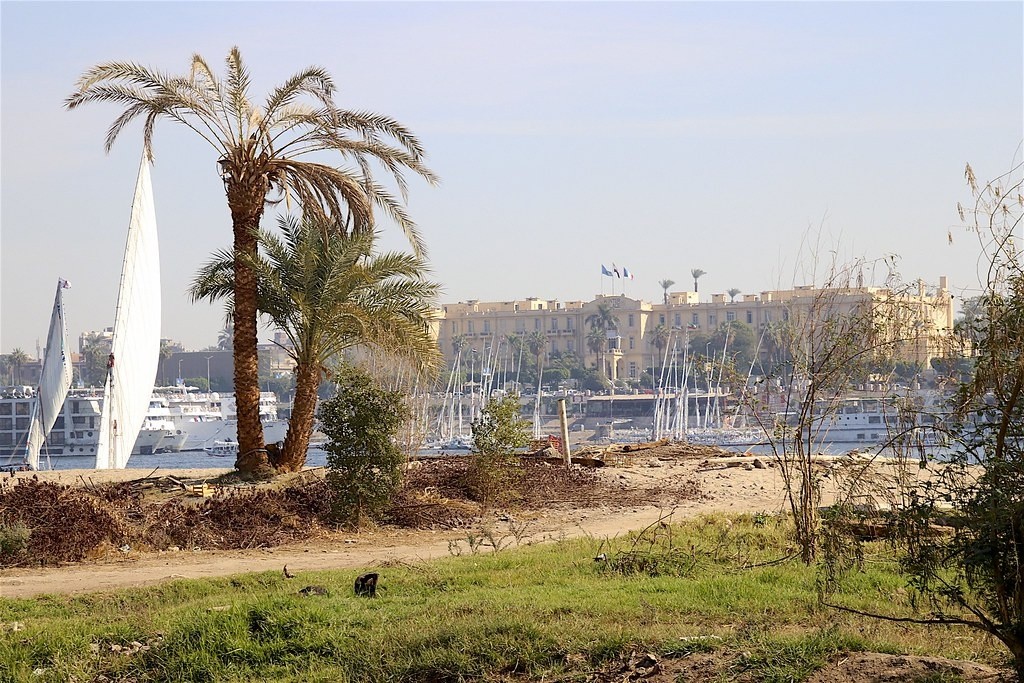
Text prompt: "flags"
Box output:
[[602, 265, 612, 276], [624, 268, 633, 280], [613, 264, 620, 278]]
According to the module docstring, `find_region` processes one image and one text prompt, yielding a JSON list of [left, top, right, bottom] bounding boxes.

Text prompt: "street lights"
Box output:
[[204, 355, 214, 393], [179, 359, 184, 378]]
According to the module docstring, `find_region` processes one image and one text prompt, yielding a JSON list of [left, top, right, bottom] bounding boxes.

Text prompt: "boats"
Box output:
[[202, 440, 239, 457], [0, 384, 289, 457], [794, 397, 948, 448]]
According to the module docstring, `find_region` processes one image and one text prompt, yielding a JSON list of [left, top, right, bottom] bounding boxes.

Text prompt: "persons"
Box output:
[[109, 353, 114, 367]]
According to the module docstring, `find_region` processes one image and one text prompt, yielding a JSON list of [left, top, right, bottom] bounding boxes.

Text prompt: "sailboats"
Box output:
[[391, 331, 555, 458], [647, 321, 806, 448]]
